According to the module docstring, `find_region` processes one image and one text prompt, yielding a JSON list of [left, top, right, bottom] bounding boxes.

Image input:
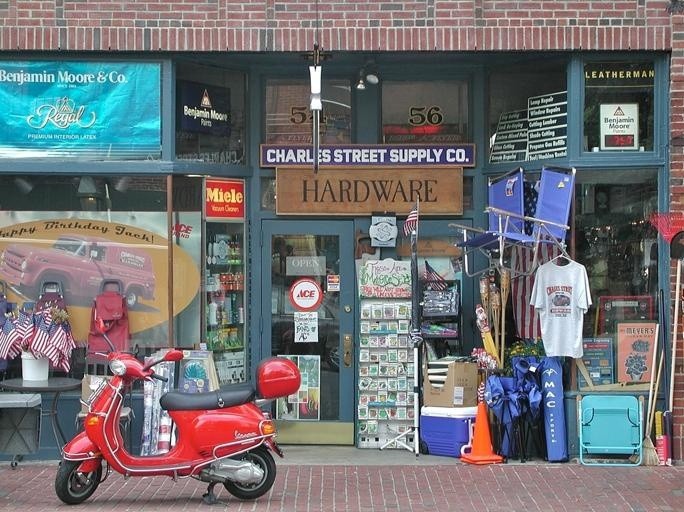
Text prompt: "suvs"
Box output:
[[1, 235, 156, 308]]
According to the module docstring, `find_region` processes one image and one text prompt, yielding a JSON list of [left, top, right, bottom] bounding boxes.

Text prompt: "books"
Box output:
[[357, 304, 414, 451]]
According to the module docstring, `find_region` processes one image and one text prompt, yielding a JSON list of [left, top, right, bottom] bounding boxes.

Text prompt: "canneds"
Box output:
[[238, 307, 243, 324]]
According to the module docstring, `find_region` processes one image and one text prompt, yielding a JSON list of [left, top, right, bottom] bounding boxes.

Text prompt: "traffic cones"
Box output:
[[461, 401, 504, 465]]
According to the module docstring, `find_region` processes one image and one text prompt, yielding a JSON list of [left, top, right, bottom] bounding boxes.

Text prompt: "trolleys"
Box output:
[[88, 278, 130, 352], [35, 280, 71, 377], [0, 281, 9, 372]]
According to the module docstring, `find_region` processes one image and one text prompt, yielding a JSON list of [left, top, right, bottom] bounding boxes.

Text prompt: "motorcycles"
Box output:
[[55, 318, 300, 505]]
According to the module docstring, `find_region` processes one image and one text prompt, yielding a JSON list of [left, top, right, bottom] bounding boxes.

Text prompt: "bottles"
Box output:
[[204, 232, 245, 349]]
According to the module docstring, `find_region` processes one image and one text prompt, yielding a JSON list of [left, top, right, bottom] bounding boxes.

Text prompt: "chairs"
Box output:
[[74, 373, 133, 456], [446, 163, 577, 277]]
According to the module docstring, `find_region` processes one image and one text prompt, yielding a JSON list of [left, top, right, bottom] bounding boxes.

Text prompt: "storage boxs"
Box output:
[[419, 362, 478, 459]]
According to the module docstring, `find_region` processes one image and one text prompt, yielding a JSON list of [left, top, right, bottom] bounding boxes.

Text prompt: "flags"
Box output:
[[423, 259, 448, 292], [403, 203, 417, 238], [0, 301, 77, 373], [509, 239, 563, 343]]
